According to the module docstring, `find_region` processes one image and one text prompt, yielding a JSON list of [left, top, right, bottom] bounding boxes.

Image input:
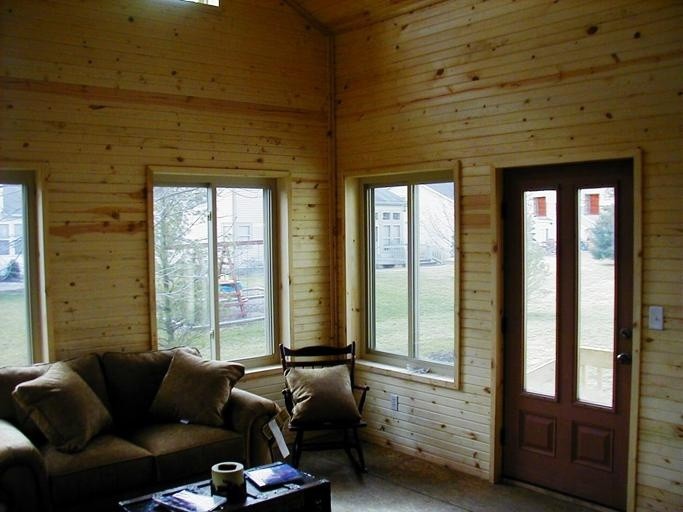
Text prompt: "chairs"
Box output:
[[278, 341, 371, 476]]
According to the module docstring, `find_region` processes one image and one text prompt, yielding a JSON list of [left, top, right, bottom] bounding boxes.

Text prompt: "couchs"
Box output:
[[0, 345, 289, 511]]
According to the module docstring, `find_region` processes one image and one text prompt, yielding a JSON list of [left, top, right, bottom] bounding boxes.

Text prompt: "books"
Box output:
[[244, 463, 305, 492], [151, 488, 228, 512]]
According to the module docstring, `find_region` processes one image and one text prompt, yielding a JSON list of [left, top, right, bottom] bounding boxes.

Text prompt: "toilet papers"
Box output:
[[211, 461, 245, 490]]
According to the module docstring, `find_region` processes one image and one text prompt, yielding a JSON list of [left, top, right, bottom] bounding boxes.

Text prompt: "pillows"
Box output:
[[147, 347, 245, 425], [284, 364, 362, 424], [12, 361, 113, 452]]
